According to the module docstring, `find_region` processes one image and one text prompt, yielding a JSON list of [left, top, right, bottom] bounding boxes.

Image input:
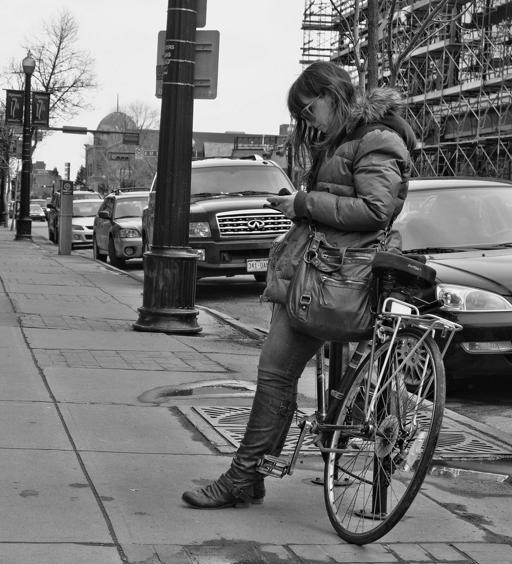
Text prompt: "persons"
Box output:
[[181, 61, 417, 510]]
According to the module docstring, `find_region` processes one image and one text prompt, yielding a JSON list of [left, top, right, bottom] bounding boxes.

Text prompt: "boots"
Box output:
[[182, 394, 300, 509]]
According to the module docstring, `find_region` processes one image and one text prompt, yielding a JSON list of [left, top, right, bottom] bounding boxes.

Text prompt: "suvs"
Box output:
[[92, 185, 150, 269], [139, 152, 304, 286], [46, 187, 103, 244]]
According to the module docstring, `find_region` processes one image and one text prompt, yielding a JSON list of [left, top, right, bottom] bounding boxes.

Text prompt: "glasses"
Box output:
[[302, 95, 325, 121]]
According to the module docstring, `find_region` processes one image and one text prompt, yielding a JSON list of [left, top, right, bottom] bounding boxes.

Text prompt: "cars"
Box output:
[[263, 172, 512, 401], [56, 198, 105, 251], [7, 196, 51, 223]]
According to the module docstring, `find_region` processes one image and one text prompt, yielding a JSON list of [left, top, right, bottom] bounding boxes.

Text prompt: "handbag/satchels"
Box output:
[[285, 227, 388, 341]]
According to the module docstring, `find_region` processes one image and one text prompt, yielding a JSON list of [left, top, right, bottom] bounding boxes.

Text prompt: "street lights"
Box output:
[[12, 47, 38, 242], [40, 184, 54, 193]]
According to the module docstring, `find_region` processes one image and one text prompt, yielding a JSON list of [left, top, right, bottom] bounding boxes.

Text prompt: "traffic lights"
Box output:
[[111, 154, 131, 162]]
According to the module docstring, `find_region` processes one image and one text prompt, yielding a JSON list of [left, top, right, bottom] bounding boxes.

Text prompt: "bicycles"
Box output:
[[251, 185, 470, 549]]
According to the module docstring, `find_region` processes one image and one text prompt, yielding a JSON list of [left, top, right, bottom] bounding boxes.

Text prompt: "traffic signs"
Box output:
[[143, 151, 158, 157]]
